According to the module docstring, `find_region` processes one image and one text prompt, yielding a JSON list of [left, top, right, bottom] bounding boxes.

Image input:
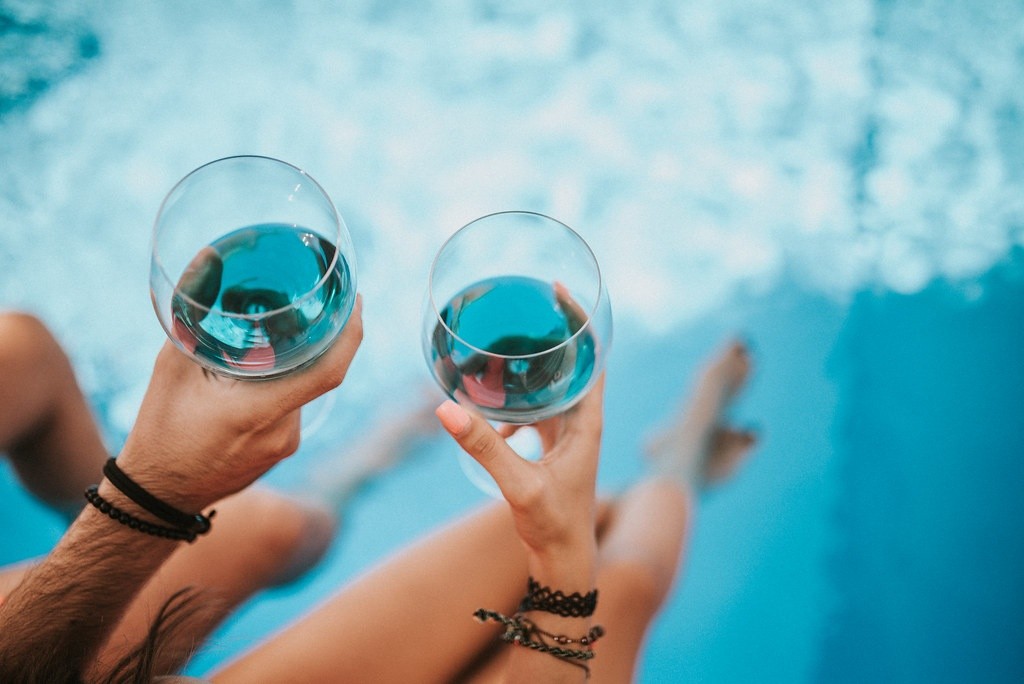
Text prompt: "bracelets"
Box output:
[[471, 607, 606, 679], [86, 490, 193, 544], [98, 454, 210, 535]]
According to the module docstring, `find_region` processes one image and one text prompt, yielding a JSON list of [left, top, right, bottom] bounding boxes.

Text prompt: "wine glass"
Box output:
[[150, 155, 358, 441], [419, 211, 614, 499]]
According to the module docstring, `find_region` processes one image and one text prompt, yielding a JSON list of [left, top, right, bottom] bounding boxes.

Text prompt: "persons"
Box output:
[[207, 280, 759, 684], [0, 248, 366, 684]]
